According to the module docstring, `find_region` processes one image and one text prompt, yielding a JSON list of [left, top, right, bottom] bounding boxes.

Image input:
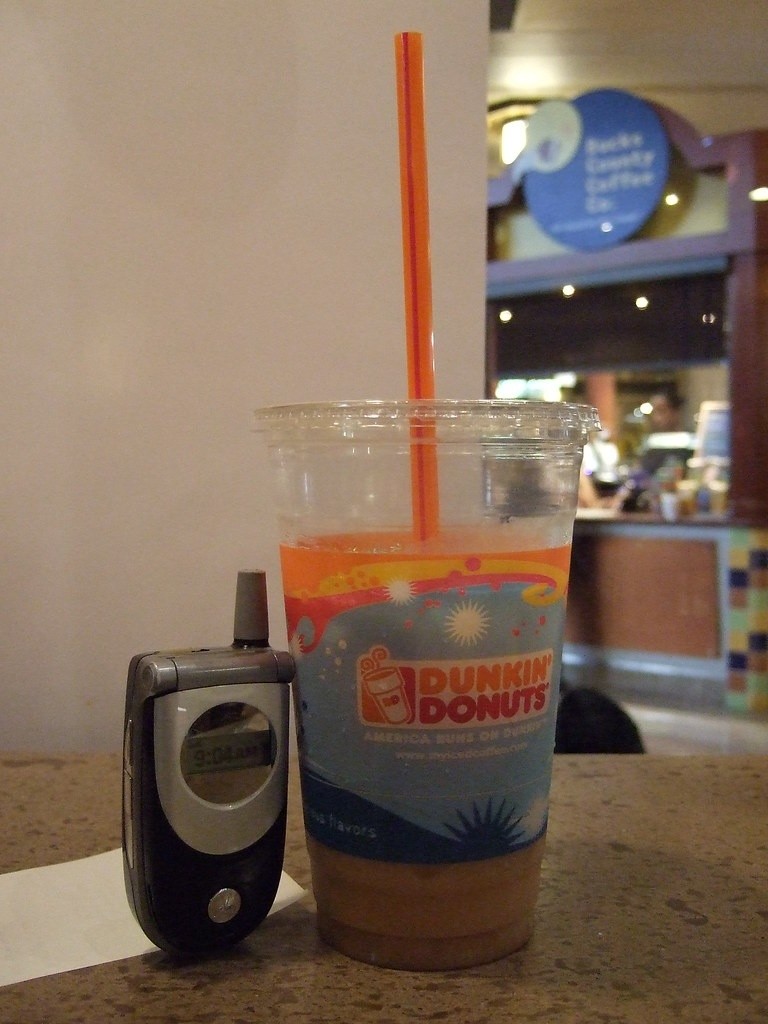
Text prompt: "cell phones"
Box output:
[[122, 567, 297, 958]]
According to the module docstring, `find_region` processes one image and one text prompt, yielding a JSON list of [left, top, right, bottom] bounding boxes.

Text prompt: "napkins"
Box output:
[[0, 847, 311, 987]]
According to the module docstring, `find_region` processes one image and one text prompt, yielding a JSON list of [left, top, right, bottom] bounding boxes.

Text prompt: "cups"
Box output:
[[253, 401, 600, 974], [659, 453, 729, 522]]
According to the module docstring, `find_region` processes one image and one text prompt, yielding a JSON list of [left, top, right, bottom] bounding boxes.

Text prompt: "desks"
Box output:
[[0, 752, 768, 1024]]
